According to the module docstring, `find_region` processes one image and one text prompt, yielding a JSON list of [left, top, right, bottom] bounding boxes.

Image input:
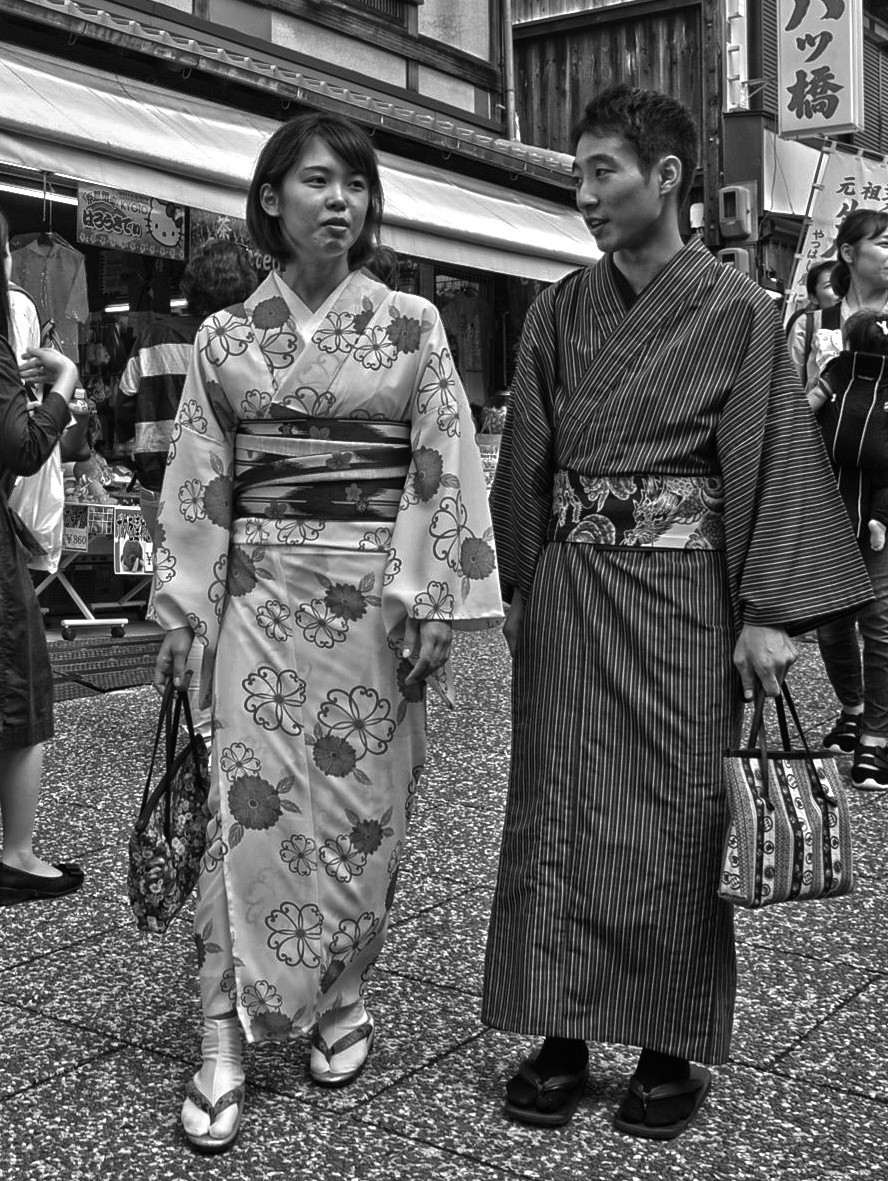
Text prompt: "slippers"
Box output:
[[506, 1058, 592, 1122], [613, 1063, 713, 1139], [180, 1066, 246, 1147], [308, 1011, 376, 1083]]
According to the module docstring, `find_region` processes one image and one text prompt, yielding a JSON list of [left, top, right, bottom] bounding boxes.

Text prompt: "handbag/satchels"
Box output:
[[127, 677, 209, 932], [716, 676, 853, 907]]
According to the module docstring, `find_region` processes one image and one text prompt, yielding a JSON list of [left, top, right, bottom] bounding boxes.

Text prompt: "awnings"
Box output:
[[0, 39, 606, 286]]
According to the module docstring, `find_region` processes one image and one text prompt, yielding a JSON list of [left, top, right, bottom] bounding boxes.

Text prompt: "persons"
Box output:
[[786, 207, 888, 790], [142, 113, 506, 1142], [487, 84, 875, 1142], [0, 209, 260, 908]]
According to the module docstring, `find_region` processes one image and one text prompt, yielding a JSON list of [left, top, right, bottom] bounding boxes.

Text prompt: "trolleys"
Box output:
[[33, 454, 153, 641]]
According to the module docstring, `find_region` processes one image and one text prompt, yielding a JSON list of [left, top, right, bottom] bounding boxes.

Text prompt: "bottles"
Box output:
[[68, 387, 88, 414]]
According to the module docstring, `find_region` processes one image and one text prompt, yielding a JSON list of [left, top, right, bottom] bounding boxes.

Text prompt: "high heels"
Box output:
[[0, 862, 85, 905]]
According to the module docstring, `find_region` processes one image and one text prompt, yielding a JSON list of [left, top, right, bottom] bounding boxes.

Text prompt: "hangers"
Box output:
[[37, 221, 53, 247]]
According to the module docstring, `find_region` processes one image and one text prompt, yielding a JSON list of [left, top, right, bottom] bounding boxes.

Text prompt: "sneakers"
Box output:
[[823, 710, 864, 754], [850, 731, 888, 790]]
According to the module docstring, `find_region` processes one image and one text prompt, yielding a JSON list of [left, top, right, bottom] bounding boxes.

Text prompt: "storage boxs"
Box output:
[[62, 500, 113, 534]]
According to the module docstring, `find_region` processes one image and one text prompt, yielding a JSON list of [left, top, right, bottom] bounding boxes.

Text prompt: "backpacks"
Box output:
[[7, 284, 92, 463]]
